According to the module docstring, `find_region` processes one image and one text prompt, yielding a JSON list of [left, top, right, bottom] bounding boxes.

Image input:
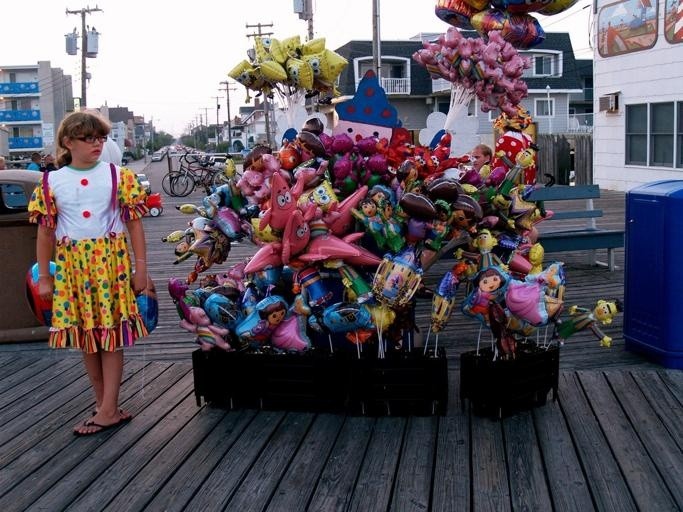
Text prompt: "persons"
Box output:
[[27, 111, 148, 439], [0, 156, 7, 170], [42, 154, 58, 173], [27, 152, 42, 171]]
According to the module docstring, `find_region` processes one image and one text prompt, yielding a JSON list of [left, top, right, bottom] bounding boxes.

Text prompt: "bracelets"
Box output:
[[134, 259, 147, 265], [38, 274, 49, 278]]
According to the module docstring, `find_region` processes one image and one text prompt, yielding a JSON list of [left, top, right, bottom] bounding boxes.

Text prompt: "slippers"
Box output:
[[92, 408, 132, 423], [73, 418, 122, 437]]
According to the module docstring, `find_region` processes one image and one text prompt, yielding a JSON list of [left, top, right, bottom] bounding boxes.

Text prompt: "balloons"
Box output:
[[24, 261, 57, 328], [164, 0, 621, 353], [130, 270, 155, 335]]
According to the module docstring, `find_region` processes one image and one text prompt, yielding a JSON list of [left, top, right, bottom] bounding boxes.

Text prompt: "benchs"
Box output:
[[527, 184, 624, 271]]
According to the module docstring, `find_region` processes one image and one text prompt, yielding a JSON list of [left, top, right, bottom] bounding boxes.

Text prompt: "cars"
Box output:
[[137, 174, 152, 195], [151, 145, 201, 161]]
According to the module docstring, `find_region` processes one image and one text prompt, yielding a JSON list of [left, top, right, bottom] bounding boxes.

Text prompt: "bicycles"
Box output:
[[162, 152, 242, 197]]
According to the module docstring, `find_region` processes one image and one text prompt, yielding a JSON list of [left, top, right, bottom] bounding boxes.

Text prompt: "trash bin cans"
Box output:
[[622, 179, 683, 370]]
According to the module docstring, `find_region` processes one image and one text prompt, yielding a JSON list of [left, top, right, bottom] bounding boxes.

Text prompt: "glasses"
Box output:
[[69, 134, 107, 143]]
